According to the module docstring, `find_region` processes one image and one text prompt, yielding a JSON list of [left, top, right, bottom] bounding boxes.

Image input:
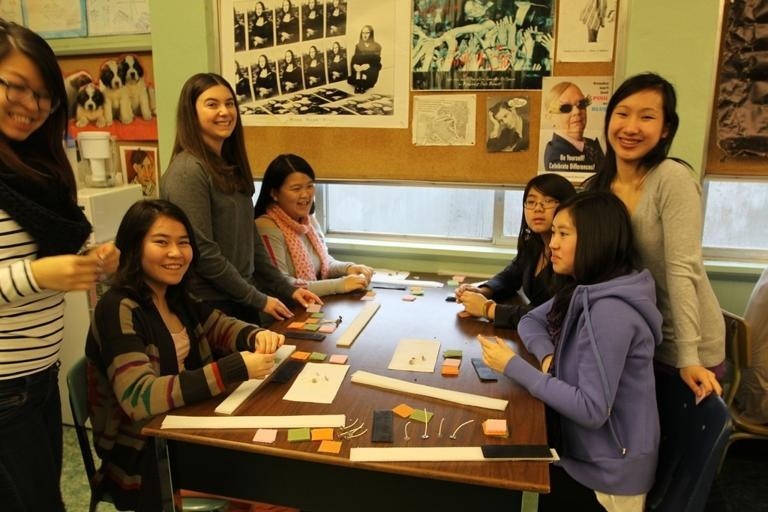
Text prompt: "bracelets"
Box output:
[[481, 300, 495, 318]]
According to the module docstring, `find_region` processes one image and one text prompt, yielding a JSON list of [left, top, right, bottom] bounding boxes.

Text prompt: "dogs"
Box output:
[[75, 82, 106, 129], [62, 70, 92, 117], [120, 56, 151, 121], [99, 59, 133, 127]]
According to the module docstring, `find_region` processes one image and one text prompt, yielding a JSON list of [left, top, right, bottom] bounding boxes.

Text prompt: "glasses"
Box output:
[[0, 76, 61, 116], [521, 196, 558, 210], [558, 97, 592, 114]]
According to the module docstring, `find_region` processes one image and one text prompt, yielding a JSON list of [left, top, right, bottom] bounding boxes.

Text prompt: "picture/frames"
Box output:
[[115, 142, 158, 203]]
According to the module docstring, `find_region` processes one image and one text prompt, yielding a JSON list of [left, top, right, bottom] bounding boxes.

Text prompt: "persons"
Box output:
[[451, 173, 577, 327], [157, 72, 323, 331], [581, 0, 606, 45], [543, 82, 603, 170], [1, 20, 124, 511], [78, 196, 287, 511], [412, 0, 554, 87], [487, 101, 529, 151], [231, 0, 352, 116], [578, 69, 726, 401], [476, 189, 665, 512], [130, 151, 155, 197], [255, 153, 374, 299], [737, 266, 767, 425], [351, 25, 382, 94]]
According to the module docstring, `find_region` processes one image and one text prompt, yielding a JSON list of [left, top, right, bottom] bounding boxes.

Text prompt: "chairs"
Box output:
[[643, 365, 733, 511], [66, 354, 230, 511], [713, 304, 768, 475]]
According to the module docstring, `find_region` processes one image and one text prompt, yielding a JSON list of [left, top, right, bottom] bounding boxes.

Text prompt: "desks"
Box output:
[[137, 280, 554, 512]]
